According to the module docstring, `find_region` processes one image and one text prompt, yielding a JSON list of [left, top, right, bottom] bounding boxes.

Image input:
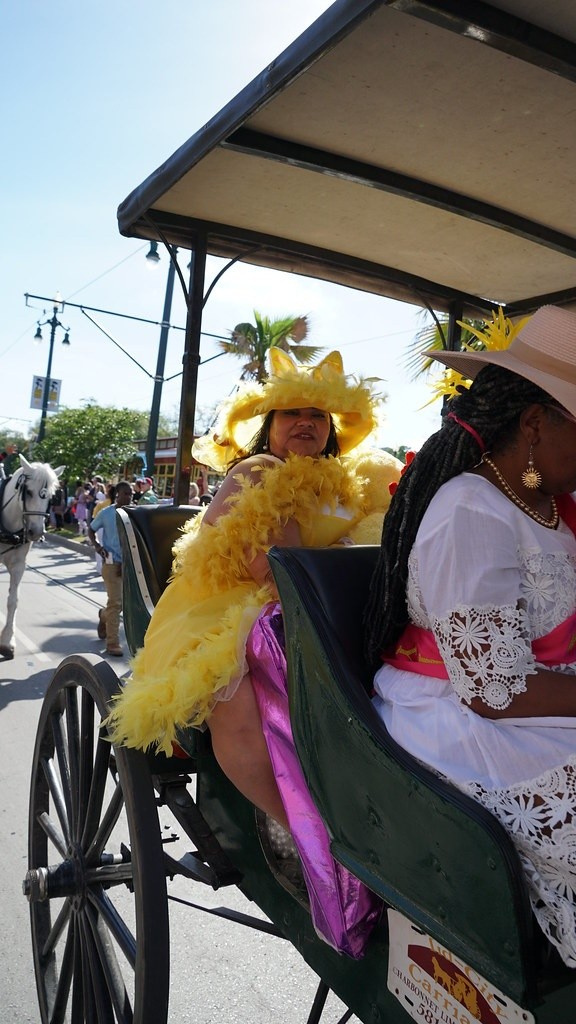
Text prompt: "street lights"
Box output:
[[145, 238, 194, 485], [34, 307, 72, 447]]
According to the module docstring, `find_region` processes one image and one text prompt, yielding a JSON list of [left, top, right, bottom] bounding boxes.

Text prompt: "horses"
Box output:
[[1, 454, 67, 661]]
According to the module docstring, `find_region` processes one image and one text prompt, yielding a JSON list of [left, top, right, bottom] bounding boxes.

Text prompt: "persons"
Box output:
[[371, 306, 575, 970], [170, 381, 360, 841], [0, 442, 219, 659]]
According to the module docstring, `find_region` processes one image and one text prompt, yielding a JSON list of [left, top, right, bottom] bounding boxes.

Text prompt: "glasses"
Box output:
[[118, 491, 132, 495]]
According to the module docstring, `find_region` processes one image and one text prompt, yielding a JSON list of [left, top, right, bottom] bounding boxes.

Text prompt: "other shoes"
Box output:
[[107, 644, 123, 656], [98, 608, 107, 640], [45, 522, 101, 575]]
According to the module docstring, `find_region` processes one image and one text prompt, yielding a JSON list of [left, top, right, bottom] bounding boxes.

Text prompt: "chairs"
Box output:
[[115, 503, 212, 763], [268, 544, 576, 1013]]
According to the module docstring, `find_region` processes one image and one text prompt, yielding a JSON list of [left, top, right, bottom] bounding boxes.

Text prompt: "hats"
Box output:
[[141, 478, 152, 485], [422, 305, 575, 421]]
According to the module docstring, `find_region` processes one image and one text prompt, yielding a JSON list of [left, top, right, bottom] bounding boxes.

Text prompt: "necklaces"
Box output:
[[478, 452, 558, 528]]
